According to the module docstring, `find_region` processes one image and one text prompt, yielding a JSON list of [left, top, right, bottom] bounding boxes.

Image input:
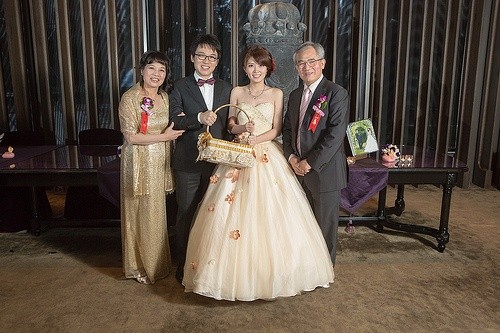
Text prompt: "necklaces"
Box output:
[[248, 84, 265, 99]]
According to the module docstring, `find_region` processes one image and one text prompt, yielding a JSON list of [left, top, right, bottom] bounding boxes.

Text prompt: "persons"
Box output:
[[355, 128, 379, 152], [117, 49, 188, 284], [166, 34, 236, 288], [282, 40, 351, 272], [180, 45, 335, 302]]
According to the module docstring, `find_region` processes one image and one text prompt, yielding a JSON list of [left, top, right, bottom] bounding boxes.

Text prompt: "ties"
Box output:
[[296, 88, 311, 155]]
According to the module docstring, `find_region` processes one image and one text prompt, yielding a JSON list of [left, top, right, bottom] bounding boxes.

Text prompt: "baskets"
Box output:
[[195, 104, 256, 167]]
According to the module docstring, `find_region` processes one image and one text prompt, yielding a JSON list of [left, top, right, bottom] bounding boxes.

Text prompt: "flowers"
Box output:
[[312, 95, 328, 116], [140, 97, 154, 115]]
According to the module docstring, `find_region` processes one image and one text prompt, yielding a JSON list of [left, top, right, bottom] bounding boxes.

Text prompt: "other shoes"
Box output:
[[175, 264, 184, 283]]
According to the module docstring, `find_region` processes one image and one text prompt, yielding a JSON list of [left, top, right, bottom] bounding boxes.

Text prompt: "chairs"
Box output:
[[79, 128, 124, 145], [0, 126, 56, 145]]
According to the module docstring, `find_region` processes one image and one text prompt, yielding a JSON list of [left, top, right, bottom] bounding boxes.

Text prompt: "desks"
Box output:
[[338, 145, 469, 253], [0, 145, 120, 227]]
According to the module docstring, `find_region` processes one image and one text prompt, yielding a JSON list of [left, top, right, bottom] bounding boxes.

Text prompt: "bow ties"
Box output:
[[197, 78, 216, 86]]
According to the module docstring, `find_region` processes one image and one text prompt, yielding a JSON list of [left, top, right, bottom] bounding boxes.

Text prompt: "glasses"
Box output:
[[295, 58, 322, 69], [194, 53, 218, 62]]
